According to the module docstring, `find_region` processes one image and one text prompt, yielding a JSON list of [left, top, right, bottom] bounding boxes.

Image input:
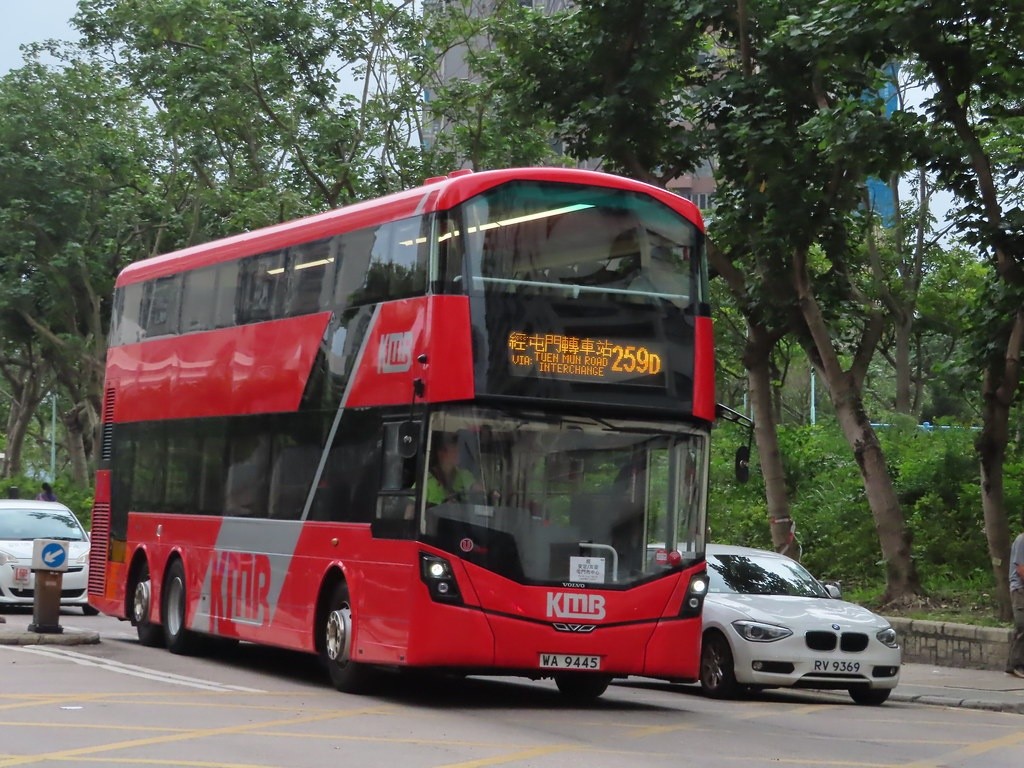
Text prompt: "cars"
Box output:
[[0, 499, 99, 616], [645, 541, 903, 707]]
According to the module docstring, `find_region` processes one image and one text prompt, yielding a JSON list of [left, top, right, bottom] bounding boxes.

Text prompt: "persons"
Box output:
[[404, 443, 501, 520], [1004, 512, 1024, 677], [36, 482, 57, 501], [572, 465, 637, 568], [626, 246, 688, 309]]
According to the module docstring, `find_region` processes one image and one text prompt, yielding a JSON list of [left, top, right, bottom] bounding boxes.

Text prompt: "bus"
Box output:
[[84, 164, 761, 701]]
